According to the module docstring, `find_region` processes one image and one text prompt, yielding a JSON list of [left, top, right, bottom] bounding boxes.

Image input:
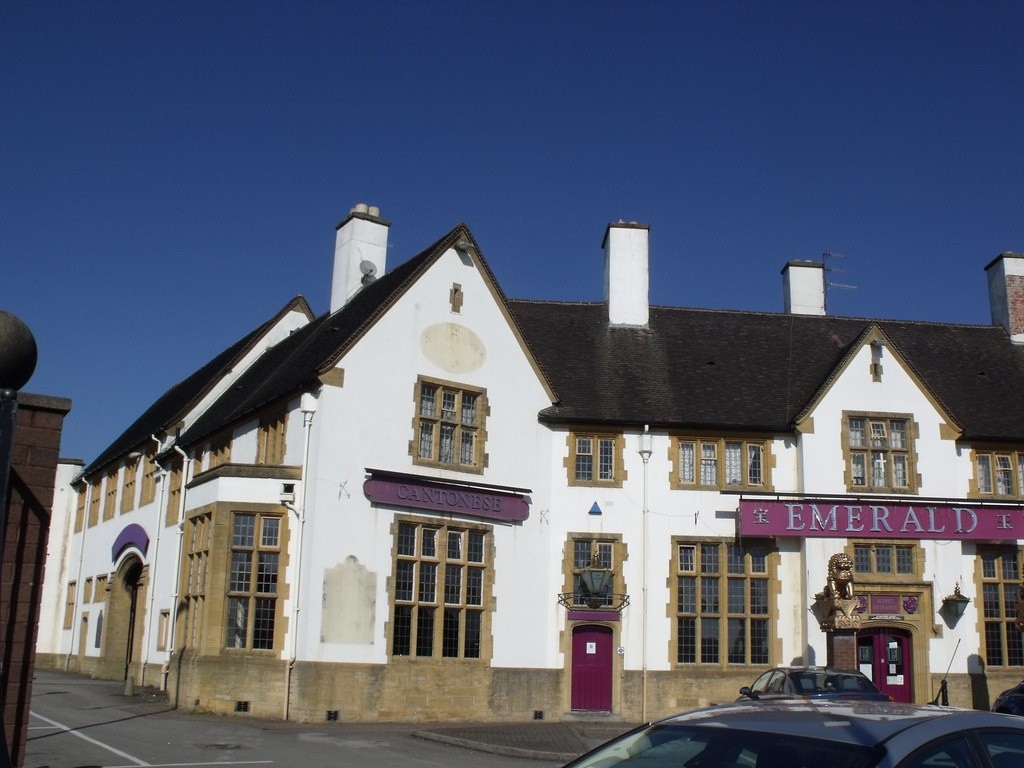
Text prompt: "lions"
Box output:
[[815, 552, 855, 601]]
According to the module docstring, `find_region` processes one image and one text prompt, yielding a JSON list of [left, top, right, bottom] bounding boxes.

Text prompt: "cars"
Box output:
[[561, 698, 1024, 768], [990, 679, 1024, 716], [733, 663, 896, 702]]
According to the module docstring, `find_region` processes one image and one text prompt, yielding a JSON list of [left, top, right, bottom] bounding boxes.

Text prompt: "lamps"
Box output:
[[871, 338, 888, 348], [454, 241, 475, 250], [941, 580, 971, 617], [580, 547, 613, 608]]
